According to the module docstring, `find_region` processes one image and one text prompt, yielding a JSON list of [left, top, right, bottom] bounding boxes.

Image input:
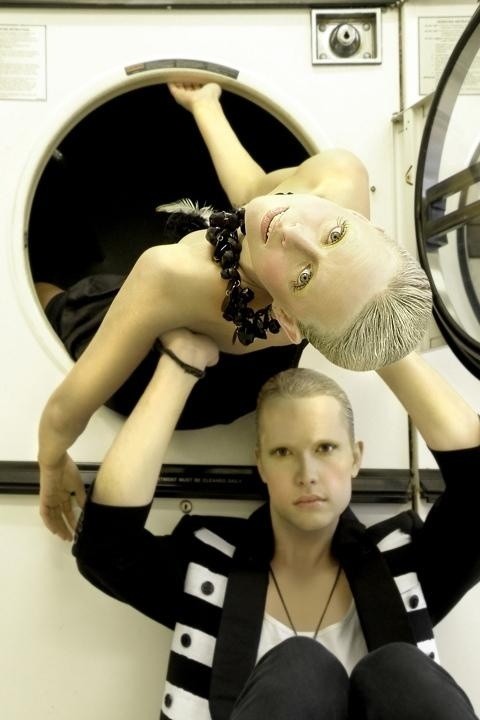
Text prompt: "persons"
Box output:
[[35, 82, 434, 540], [71, 329, 480, 719]]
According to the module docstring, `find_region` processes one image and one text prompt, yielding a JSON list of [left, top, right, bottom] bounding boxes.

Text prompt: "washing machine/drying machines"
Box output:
[[0, 0, 479, 498]]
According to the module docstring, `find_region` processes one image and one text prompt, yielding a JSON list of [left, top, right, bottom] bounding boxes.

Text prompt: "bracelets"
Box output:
[[154, 338, 206, 380]]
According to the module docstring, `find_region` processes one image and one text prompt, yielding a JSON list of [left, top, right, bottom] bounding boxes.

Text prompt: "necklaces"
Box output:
[[269, 564, 341, 640], [207, 193, 294, 345]]
